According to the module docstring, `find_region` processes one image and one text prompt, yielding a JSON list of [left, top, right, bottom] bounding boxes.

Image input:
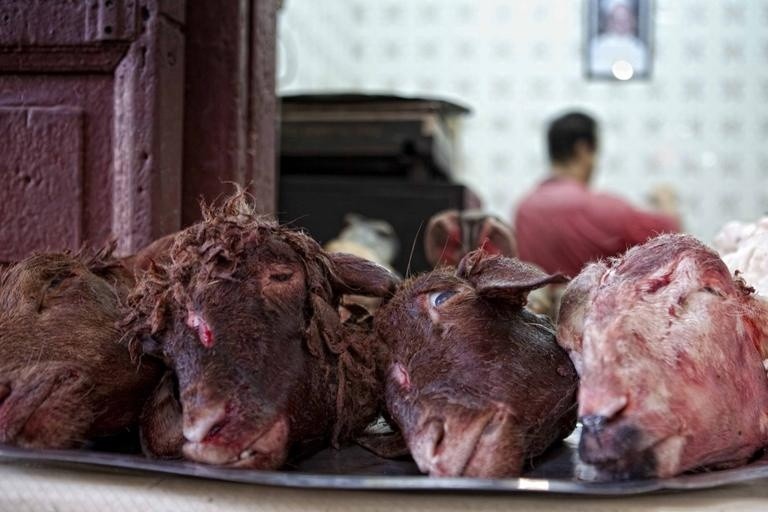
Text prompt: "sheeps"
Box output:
[[351, 220, 578, 478], [112, 173, 404, 471], [546, 228, 762, 483], [0, 235, 165, 453]]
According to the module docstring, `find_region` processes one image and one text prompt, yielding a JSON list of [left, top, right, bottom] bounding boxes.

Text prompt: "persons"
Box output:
[[515, 111, 681, 290], [592, 1, 648, 73]]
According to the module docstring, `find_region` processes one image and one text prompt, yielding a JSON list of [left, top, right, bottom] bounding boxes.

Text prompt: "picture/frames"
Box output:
[[580, 0, 658, 84]]
